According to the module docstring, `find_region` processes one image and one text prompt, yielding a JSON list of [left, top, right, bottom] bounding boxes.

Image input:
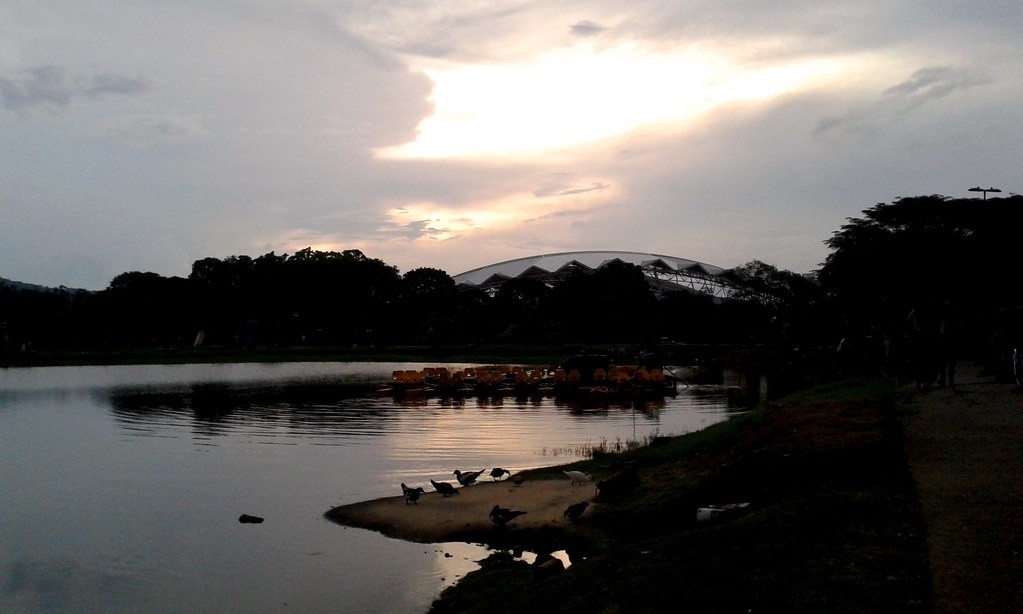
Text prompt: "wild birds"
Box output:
[[431, 480, 459, 497], [401, 482, 425, 505], [562, 469, 595, 486], [489, 505, 528, 526], [453, 469, 485, 486], [489, 468, 511, 482]]
